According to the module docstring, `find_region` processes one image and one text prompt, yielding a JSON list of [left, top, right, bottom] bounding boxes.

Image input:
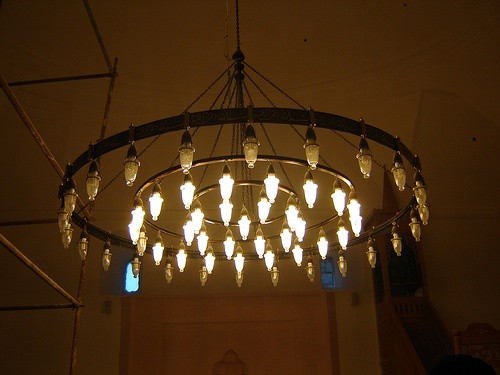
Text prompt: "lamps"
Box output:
[[59, 46, 431, 290]]
